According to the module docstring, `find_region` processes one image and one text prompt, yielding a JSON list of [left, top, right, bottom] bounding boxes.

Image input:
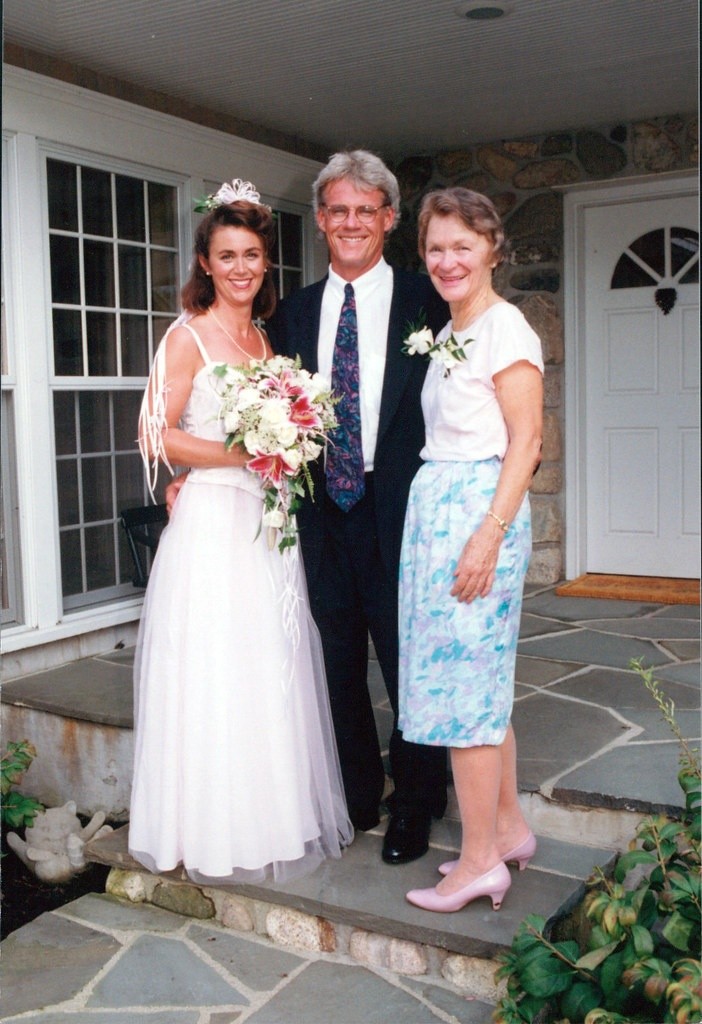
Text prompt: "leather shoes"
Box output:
[[350, 774, 387, 833], [380, 790, 434, 864]]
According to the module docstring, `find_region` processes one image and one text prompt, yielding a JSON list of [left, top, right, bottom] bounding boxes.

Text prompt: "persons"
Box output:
[[398, 186, 545, 913], [166, 148, 451, 867], [128, 179, 352, 887]]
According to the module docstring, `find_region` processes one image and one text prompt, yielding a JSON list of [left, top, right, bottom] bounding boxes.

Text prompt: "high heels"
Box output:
[[406, 861, 513, 913], [439, 827, 539, 876]]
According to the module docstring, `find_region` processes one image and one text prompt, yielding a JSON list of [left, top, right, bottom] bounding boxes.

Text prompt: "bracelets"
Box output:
[[488, 511, 510, 532]]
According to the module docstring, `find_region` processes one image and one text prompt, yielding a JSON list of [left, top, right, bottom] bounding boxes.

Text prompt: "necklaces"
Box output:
[[208, 305, 268, 360]]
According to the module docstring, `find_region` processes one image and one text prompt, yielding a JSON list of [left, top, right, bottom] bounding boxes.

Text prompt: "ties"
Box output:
[[324, 284, 366, 513]]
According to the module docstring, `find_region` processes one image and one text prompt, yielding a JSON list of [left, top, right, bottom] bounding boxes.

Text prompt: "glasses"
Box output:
[[319, 202, 390, 225]]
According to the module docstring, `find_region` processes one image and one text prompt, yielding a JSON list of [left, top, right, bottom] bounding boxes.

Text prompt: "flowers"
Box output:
[[389, 310, 475, 377], [207, 354, 345, 557]]
